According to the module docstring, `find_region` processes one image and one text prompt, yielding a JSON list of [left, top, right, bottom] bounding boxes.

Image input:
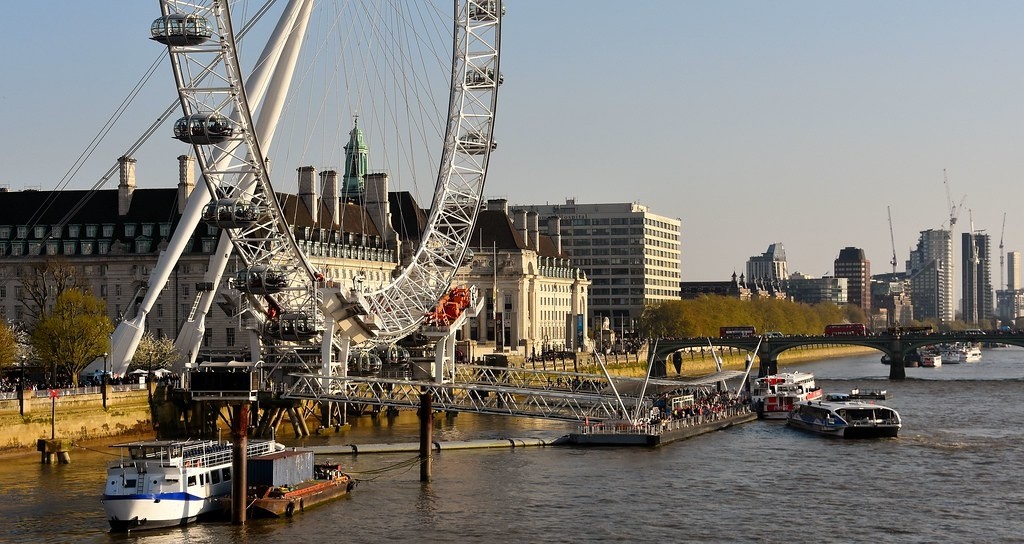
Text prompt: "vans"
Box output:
[[965, 329, 986, 337], [765, 331, 785, 340]]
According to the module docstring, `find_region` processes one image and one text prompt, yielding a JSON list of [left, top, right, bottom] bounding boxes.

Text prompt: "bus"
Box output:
[[823, 322, 866, 338], [719, 325, 756, 340], [887, 326, 934, 336]]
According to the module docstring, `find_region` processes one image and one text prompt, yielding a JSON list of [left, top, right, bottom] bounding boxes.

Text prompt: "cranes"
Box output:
[[943, 168, 969, 232], [998, 213, 1007, 291], [904, 257, 938, 303], [967, 206, 981, 328], [887, 205, 898, 282]]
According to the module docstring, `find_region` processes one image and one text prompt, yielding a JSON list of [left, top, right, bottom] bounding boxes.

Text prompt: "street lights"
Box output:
[[18, 352, 27, 417], [146, 350, 152, 401], [101, 350, 109, 409]]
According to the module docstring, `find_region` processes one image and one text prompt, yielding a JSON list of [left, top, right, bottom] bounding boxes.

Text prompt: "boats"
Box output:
[[826, 393, 851, 402], [784, 396, 902, 440], [209, 461, 362, 520], [849, 388, 887, 400], [99, 427, 287, 535], [750, 366, 824, 421]]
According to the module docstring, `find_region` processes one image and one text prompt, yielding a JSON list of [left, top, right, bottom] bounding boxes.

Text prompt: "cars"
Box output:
[[526, 350, 577, 365], [881, 341, 1011, 368]]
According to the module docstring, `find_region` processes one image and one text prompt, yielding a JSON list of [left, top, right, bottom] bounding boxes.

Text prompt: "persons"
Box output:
[[637, 388, 763, 433], [548, 377, 609, 391], [0, 373, 180, 392], [598, 328, 1024, 356]]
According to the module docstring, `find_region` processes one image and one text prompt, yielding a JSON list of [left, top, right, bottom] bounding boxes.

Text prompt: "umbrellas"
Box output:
[[131, 369, 148, 373], [154, 368, 172, 373]]
